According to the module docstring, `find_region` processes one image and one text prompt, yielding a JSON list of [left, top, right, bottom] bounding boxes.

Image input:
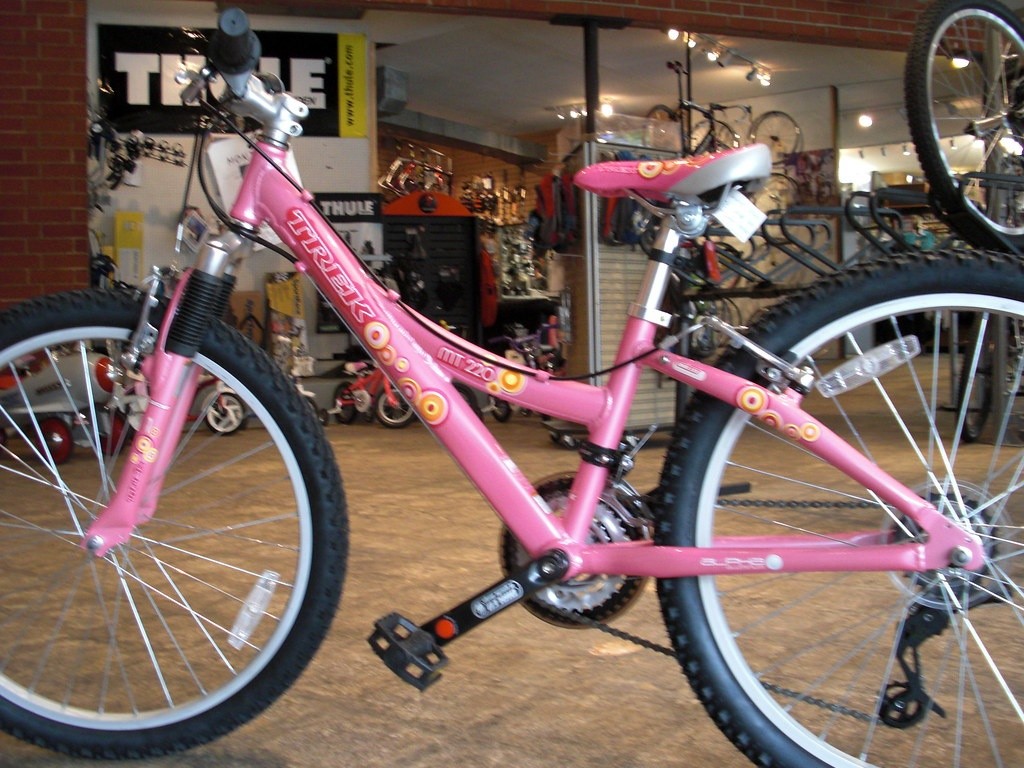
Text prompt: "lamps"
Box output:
[[717, 51, 732, 68], [948, 138, 957, 149], [859, 148, 866, 160], [699, 46, 715, 63], [660, 28, 679, 40], [747, 66, 758, 81], [557, 106, 587, 119], [902, 143, 910, 156], [881, 145, 887, 157]]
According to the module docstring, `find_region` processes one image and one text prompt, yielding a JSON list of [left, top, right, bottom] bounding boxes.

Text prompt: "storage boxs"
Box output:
[[230, 292, 263, 347], [114, 210, 144, 287], [263, 271, 312, 378]]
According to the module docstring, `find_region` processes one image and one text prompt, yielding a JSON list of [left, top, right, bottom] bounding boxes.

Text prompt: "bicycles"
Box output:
[[1, 7, 1024, 768], [480, 322, 563, 422], [630, 55, 806, 357], [898, 1, 1023, 252], [314, 350, 417, 429], [377, 140, 541, 297]]
